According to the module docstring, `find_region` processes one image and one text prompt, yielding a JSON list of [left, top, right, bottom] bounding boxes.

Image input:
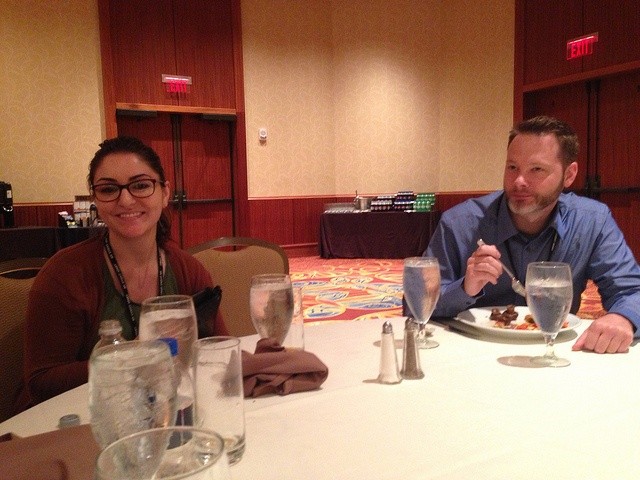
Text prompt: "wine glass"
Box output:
[[249, 274, 294, 346], [139, 294, 200, 429], [87, 340, 177, 480], [525, 262, 574, 369], [401, 256, 441, 351]]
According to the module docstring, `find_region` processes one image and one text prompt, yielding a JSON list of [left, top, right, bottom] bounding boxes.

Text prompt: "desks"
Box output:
[[319, 208, 441, 260]]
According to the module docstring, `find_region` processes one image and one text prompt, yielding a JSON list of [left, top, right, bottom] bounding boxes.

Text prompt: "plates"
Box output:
[[454, 306, 583, 337]]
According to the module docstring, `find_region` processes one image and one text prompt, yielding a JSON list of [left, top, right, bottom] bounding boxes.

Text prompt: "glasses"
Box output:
[[91, 179, 164, 202]]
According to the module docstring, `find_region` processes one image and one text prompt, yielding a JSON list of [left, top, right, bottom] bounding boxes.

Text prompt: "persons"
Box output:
[[15, 136, 231, 414], [402, 115, 640, 353]]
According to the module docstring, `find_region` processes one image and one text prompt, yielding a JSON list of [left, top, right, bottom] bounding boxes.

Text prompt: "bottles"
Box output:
[[375, 320, 403, 386], [90, 319, 129, 369], [90, 204, 98, 227], [370, 191, 436, 213], [399, 317, 426, 380]]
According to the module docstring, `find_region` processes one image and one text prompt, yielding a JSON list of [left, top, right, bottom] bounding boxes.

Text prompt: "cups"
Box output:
[[193, 335, 244, 468], [273, 288, 305, 352], [93, 425, 228, 480]]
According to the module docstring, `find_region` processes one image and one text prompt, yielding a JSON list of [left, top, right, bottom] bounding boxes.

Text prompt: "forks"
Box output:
[[477, 238, 525, 298]]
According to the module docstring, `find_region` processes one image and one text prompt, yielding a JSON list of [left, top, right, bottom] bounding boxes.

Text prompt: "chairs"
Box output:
[[0, 267, 44, 419], [191, 234, 294, 336]]
